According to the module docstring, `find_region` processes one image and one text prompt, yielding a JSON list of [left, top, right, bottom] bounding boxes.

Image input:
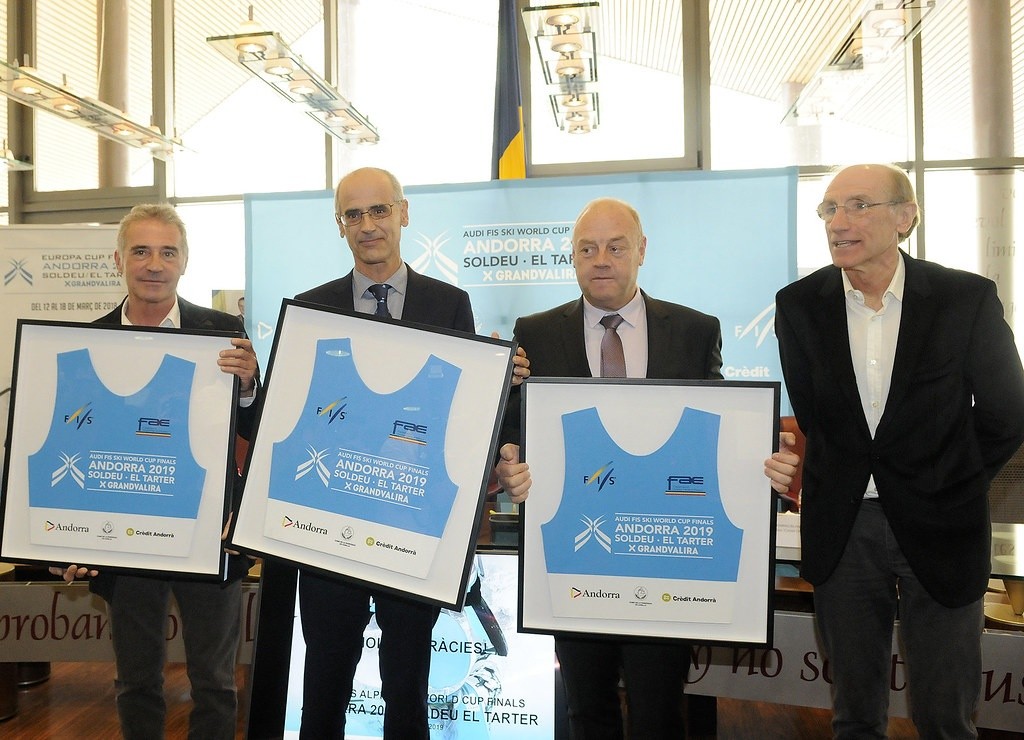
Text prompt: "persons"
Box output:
[[44, 202, 263, 740], [223, 167, 529, 740], [236, 298, 245, 322], [774, 162, 1024, 740], [495, 200, 800, 740]]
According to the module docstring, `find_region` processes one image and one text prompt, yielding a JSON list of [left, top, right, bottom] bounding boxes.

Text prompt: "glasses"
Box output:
[[817, 198, 903, 221], [337, 200, 400, 226]]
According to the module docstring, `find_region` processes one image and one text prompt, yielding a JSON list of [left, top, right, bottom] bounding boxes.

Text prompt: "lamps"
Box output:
[[0, 1, 200, 172], [779, 0, 947, 128], [206, 0, 380, 148], [521, 0, 602, 135]]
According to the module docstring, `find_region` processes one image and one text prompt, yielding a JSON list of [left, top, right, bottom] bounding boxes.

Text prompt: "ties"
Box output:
[[600, 313, 628, 381], [368, 284, 393, 317]]
